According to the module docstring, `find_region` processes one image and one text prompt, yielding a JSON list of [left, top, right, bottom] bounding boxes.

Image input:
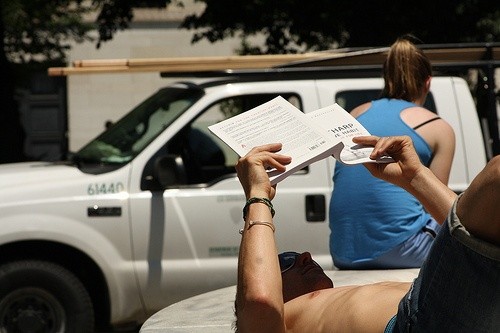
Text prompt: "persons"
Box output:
[[328, 39, 456, 272], [236, 133, 500, 333]]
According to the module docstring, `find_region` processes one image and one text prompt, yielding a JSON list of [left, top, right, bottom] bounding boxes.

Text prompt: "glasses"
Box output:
[[277, 251, 322, 273]]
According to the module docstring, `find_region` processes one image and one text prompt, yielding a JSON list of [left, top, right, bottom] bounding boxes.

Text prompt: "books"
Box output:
[[206, 94, 397, 188]]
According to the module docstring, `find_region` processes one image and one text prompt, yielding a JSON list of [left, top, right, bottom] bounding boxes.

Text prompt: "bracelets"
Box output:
[[239, 222, 276, 233], [242, 197, 275, 221]]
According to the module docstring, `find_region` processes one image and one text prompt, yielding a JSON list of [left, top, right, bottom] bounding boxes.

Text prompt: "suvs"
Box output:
[[0, 61, 500, 333]]
